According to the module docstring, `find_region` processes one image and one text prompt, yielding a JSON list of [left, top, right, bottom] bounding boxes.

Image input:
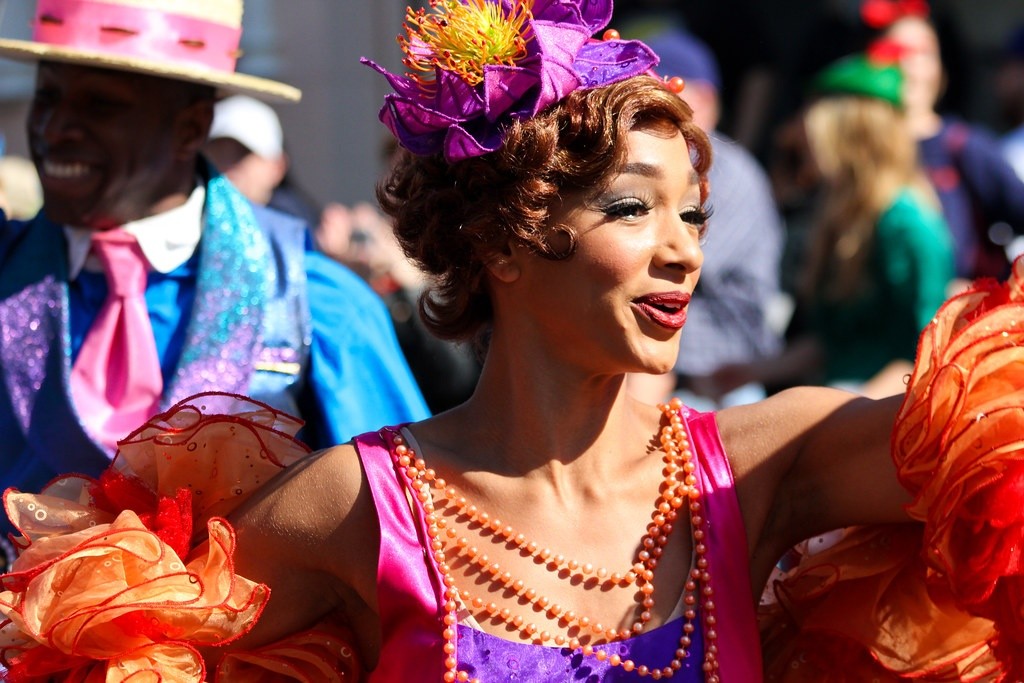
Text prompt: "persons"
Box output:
[[629, 1, 1023, 413], [0, 0, 477, 573], [184, 76, 923, 683]]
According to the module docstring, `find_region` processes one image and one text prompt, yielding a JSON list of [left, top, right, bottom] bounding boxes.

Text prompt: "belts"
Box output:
[[674, 360, 765, 400]]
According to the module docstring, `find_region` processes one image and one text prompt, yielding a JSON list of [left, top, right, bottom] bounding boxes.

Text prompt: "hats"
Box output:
[[643, 26, 721, 90], [208, 94, 283, 158], [818, 51, 907, 107], [0, 0, 301, 104]]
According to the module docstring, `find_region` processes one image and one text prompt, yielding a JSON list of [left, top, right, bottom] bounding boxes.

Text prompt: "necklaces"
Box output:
[[387, 399, 720, 681]]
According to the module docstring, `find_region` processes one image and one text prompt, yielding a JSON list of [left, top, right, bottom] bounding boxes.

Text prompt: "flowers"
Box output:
[[359, 1, 662, 161]]
